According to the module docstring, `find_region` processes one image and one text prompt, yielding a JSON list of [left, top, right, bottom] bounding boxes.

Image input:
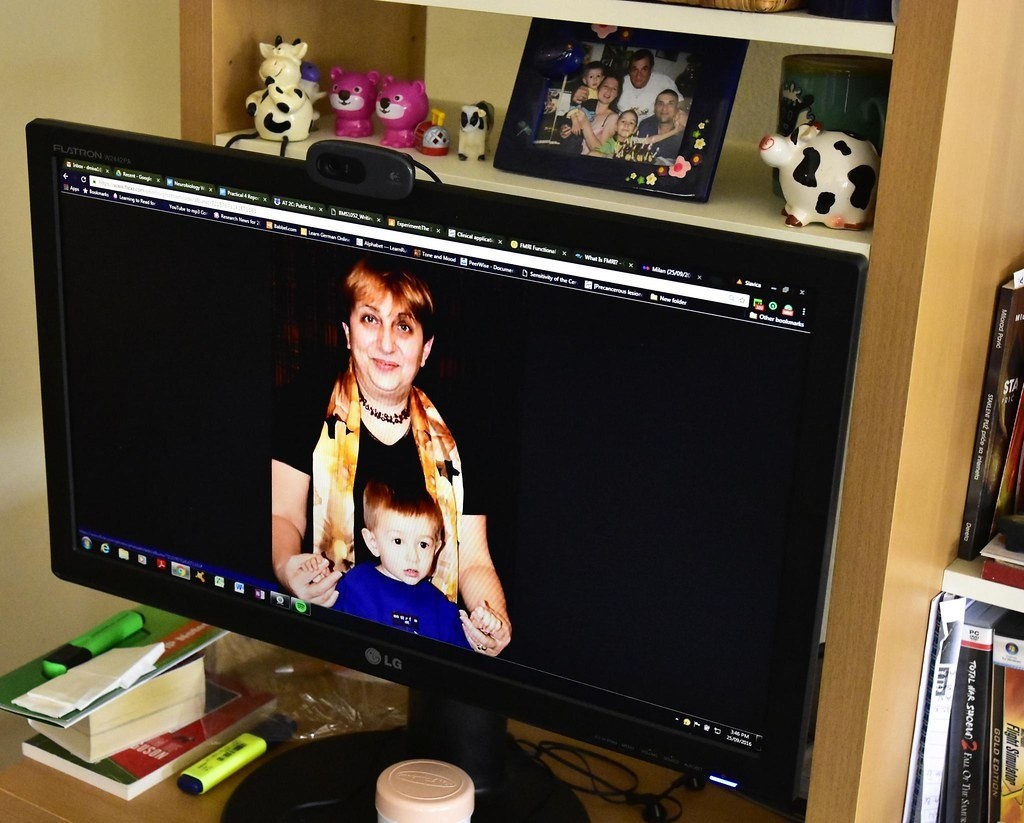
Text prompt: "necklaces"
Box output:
[[357, 390, 410, 424]]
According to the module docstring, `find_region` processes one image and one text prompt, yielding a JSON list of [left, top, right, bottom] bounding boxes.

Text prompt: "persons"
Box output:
[[301, 476, 502, 650], [557, 48, 692, 164], [270, 254, 511, 657]]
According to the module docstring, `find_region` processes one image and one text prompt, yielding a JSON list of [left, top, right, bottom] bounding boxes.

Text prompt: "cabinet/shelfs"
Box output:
[[0, 1, 1024, 822]]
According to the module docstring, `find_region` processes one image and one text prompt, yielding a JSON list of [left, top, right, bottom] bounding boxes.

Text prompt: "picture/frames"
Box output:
[[654, 48, 680, 63], [493, 16, 750, 202]]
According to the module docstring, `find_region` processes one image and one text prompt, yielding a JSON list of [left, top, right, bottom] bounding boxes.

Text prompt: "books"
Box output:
[[903, 593, 1023, 823], [0, 604, 277, 801], [957, 273, 1024, 567]]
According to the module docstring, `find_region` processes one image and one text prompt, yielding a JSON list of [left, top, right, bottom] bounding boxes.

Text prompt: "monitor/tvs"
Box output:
[[24, 117, 867, 823]]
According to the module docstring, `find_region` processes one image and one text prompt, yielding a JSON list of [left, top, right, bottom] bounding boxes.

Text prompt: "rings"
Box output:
[[478, 645, 487, 651]]
